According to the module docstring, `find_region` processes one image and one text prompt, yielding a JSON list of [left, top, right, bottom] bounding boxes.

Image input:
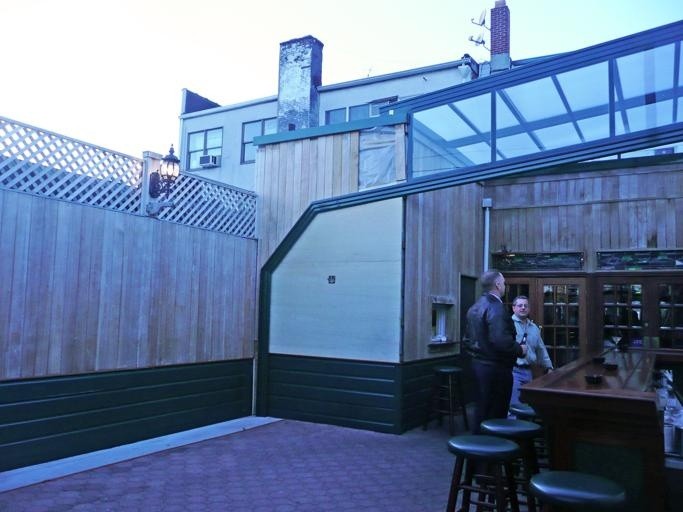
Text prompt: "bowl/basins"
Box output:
[[604, 362, 618, 371], [585, 375, 604, 384], [591, 357, 604, 364]]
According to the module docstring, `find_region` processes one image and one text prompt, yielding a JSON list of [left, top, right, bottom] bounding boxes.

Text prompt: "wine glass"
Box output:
[[613, 337, 621, 351]]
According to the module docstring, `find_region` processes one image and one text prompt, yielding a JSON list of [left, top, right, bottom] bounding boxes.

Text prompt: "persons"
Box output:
[[464, 268, 528, 484], [505, 293, 554, 420]]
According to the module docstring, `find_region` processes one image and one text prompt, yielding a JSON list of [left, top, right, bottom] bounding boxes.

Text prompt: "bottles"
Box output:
[[518, 332, 529, 358]]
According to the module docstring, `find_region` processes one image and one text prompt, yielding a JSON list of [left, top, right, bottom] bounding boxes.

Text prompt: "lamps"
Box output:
[[148, 143, 181, 200]]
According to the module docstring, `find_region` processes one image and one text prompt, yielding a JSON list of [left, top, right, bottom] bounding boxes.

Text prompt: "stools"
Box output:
[[509, 403, 553, 471], [422, 366, 468, 437], [529, 470, 626, 511], [446, 436, 522, 512], [477, 418, 548, 512]]
